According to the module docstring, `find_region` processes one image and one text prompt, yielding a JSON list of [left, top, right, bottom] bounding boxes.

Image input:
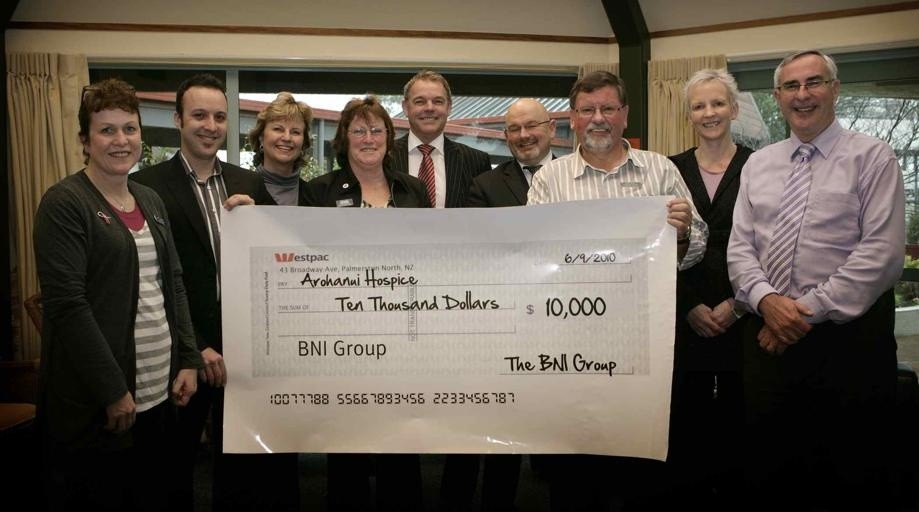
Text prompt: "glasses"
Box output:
[[81, 86, 135, 102], [571, 103, 624, 117], [782, 80, 830, 89], [348, 128, 386, 137]]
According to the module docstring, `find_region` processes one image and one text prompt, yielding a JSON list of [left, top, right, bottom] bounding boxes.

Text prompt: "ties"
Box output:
[[767, 143, 816, 297], [417, 144, 436, 208]]
